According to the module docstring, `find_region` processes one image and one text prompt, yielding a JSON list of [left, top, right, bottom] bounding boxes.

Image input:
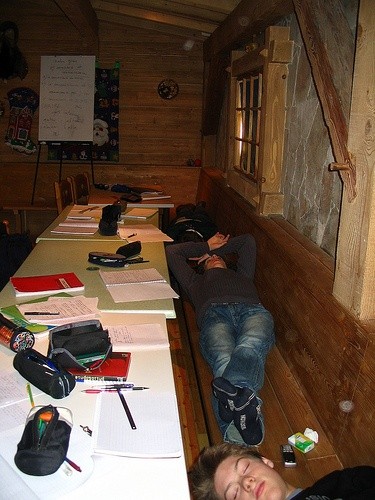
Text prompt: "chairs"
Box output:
[[54, 176, 75, 214], [69, 171, 90, 205]]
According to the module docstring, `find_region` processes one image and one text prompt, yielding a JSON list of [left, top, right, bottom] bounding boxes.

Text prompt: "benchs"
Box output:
[[162, 166, 375, 500], [3, 204, 65, 234]]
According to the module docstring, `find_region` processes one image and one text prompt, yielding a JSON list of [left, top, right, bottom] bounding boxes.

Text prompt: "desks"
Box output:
[[84, 184, 175, 226], [0, 240, 177, 319], [0, 313, 193, 500], [35, 202, 160, 241]]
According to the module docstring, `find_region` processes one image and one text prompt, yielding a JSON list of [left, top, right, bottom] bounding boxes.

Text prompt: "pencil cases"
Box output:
[[46, 320, 112, 372], [88, 252, 126, 267], [0, 312, 36, 353], [112, 201, 127, 213], [13, 347, 76, 400], [14, 404, 73, 476], [117, 194, 143, 203], [116, 241, 141, 258], [99, 204, 118, 236]]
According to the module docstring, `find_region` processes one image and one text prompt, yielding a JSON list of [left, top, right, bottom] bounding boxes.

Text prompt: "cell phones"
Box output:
[[281, 444, 297, 467]]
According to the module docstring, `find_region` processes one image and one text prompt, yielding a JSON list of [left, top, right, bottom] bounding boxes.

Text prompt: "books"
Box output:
[[140, 193, 171, 200], [124, 207, 158, 220], [51, 203, 102, 234], [0, 291, 99, 338], [116, 223, 174, 242], [10, 272, 85, 297], [99, 268, 180, 303]]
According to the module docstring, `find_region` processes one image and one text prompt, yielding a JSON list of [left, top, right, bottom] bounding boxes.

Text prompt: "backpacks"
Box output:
[[165, 216, 208, 249], [175, 201, 205, 217]]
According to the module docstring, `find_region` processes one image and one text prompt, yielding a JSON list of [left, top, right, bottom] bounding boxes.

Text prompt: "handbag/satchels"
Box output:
[[182, 208, 216, 239]]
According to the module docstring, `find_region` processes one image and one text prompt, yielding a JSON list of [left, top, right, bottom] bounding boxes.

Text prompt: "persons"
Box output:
[[166, 231, 277, 447], [189, 443, 375, 500], [164, 203, 210, 245]]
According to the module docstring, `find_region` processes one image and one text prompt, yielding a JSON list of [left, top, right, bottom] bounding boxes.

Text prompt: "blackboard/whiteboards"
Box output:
[[37, 56, 94, 142]]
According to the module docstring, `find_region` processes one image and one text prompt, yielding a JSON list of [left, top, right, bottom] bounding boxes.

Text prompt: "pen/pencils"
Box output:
[[81, 384, 149, 394], [26, 383, 35, 408], [117, 387, 137, 430], [65, 457, 82, 473]]
